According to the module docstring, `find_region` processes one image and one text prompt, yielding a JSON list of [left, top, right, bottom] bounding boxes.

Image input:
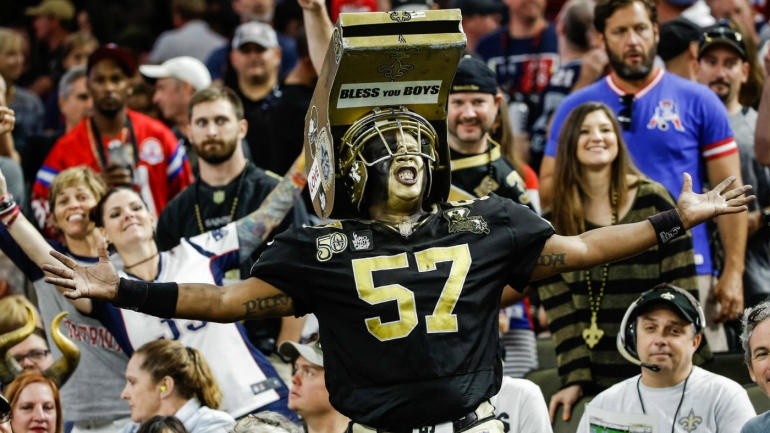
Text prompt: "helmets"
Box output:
[[342, 106, 442, 216]]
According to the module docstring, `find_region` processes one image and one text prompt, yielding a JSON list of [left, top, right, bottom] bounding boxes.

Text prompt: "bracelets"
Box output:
[[0, 193, 16, 219], [648, 209, 686, 248], [760, 207, 770, 229], [4, 209, 18, 231]]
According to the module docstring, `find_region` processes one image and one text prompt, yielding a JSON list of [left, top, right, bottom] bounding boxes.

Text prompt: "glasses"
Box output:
[[700, 27, 742, 47], [14, 349, 51, 362], [241, 46, 267, 51]]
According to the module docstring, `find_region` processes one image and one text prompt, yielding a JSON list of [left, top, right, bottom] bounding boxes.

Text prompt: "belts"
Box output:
[[378, 411, 477, 433]]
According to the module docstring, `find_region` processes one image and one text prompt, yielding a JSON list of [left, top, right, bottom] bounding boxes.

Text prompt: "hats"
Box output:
[[86, 44, 137, 77], [26, 0, 75, 19], [139, 56, 212, 91], [449, 55, 497, 95], [636, 286, 701, 332], [231, 21, 278, 49], [277, 339, 324, 367], [697, 23, 747, 60]]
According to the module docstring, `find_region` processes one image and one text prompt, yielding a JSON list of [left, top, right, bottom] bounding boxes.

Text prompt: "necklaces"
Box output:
[[486, 140, 492, 187], [125, 252, 158, 269], [575, 191, 618, 349], [91, 115, 139, 170], [193, 158, 249, 234]]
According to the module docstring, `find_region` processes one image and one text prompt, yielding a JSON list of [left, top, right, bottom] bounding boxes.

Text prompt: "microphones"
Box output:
[[616, 333, 661, 372]]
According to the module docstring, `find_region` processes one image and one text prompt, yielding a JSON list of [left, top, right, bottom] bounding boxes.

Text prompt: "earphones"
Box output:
[[161, 385, 167, 392]]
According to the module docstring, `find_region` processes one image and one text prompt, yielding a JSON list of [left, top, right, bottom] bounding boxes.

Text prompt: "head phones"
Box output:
[[619, 284, 706, 355]]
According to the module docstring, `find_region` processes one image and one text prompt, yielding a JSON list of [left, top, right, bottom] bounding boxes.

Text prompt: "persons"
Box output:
[[0, 0, 770, 433]]
